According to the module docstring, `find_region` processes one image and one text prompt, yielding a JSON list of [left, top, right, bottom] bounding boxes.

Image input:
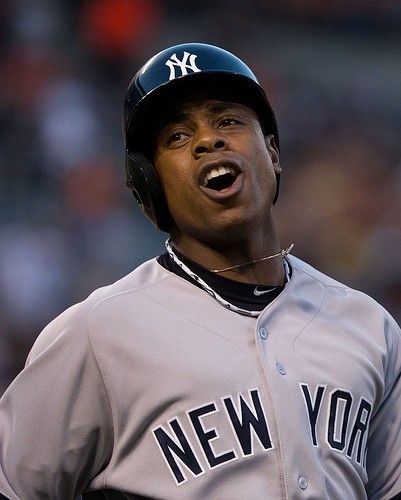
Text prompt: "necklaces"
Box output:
[[208, 242, 295, 274]]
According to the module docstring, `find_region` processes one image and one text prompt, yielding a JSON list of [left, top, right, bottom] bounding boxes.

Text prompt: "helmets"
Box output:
[[119, 42, 284, 226]]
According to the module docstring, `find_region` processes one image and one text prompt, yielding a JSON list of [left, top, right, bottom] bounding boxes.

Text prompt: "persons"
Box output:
[[0, 39, 400, 500]]
